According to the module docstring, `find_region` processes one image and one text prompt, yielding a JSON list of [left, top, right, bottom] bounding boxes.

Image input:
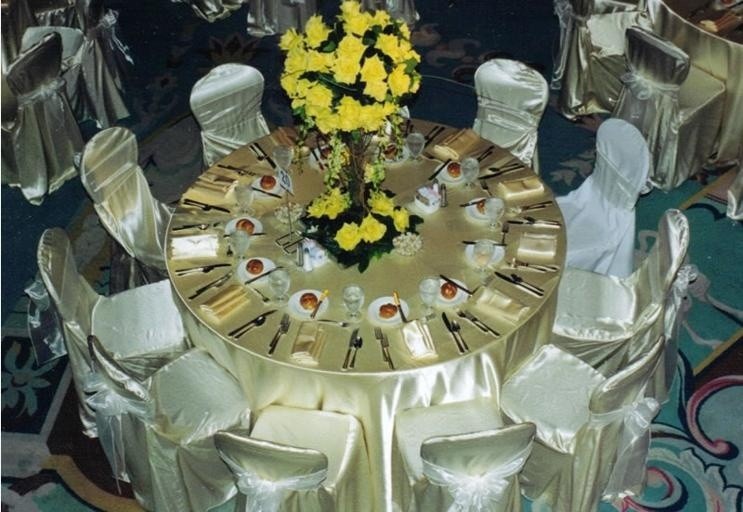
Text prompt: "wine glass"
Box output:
[[228, 228, 249, 264], [343, 282, 364, 322], [417, 278, 442, 320]]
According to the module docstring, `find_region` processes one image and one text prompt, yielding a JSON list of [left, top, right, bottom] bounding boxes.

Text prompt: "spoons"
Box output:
[[316, 316, 351, 329], [349, 336, 362, 367], [449, 318, 469, 350]]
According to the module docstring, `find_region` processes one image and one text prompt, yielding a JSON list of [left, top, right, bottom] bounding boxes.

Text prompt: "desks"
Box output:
[[164, 116, 568, 510]]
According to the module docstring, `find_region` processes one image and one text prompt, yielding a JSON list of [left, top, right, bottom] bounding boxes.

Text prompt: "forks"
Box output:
[[454, 306, 487, 333], [373, 326, 387, 363], [380, 331, 395, 370]]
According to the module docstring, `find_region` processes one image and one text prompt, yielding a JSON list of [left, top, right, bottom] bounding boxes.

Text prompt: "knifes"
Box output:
[[338, 327, 359, 369], [464, 310, 500, 337], [441, 311, 464, 355], [391, 289, 408, 324]]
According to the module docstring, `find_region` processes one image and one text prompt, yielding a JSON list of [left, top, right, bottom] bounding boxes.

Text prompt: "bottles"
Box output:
[[301, 247, 313, 273], [294, 242, 302, 265]]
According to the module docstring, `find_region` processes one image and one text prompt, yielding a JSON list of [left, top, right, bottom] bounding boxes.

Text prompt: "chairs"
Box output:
[[79, 125, 177, 294], [86, 334, 250, 511], [552, 207, 709, 404], [213, 403, 373, 512], [499, 333, 668, 511], [550, 0, 743, 194], [188, 62, 270, 167], [553, 117, 652, 281], [36, 225, 194, 439], [187, 0, 427, 43], [472, 56, 550, 178], [388, 394, 536, 511], [1, 3, 136, 209]]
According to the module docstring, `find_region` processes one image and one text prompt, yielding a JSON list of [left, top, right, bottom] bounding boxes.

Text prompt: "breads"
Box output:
[[448, 162, 460, 176], [321, 147, 331, 158], [261, 175, 276, 189], [246, 260, 263, 274], [478, 199, 485, 214], [300, 294, 318, 309], [441, 283, 457, 299], [236, 219, 254, 235], [380, 304, 396, 318]]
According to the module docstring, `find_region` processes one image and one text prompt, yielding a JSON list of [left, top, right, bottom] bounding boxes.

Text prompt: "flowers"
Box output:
[[275, 0, 430, 276]]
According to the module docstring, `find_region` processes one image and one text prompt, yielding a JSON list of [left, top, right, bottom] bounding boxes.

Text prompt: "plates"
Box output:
[[383, 116, 562, 307], [368, 296, 411, 326], [172, 140, 334, 250], [175, 257, 330, 371]]
